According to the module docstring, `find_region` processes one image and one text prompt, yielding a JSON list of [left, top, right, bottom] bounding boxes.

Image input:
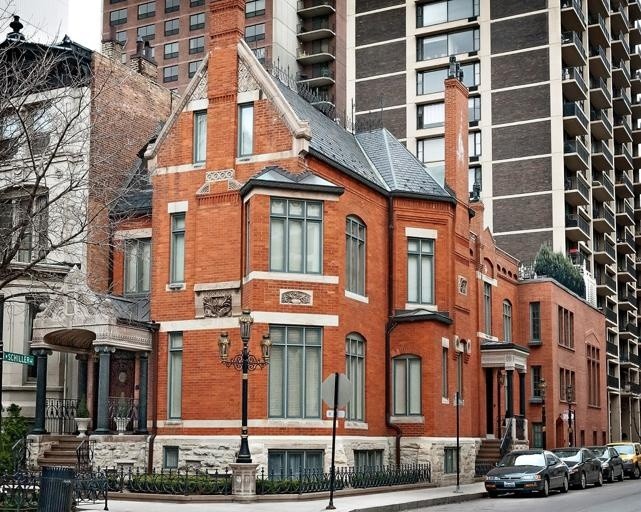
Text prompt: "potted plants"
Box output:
[[75, 393, 92, 438], [112, 393, 132, 434]]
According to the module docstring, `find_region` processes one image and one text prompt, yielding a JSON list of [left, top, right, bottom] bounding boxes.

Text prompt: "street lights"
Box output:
[[538, 378, 548, 451], [217, 308, 272, 463], [566, 385, 573, 448]]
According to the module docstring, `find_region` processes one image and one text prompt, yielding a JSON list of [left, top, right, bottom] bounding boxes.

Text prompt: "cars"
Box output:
[[484, 442, 641, 497]]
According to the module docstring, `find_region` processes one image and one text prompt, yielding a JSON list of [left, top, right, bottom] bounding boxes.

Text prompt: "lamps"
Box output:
[[498, 374, 509, 387]]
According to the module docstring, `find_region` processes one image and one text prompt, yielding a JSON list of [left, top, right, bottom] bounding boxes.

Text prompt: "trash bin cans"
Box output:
[[37, 465, 75, 512]]
